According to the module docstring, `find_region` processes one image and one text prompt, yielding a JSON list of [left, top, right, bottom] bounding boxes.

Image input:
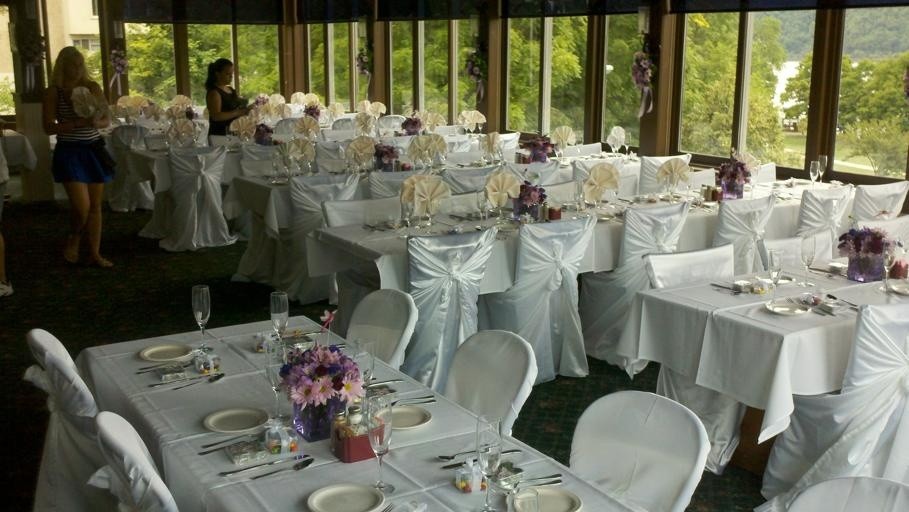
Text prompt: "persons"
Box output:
[[0, 142, 13, 298], [43, 47, 115, 267], [205, 59, 257, 147]]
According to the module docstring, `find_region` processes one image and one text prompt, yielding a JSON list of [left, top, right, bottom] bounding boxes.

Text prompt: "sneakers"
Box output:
[[0, 281, 15, 299]]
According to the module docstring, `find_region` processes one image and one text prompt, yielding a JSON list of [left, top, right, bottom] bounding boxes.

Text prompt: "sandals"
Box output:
[[87, 256, 114, 269], [62, 245, 80, 265]]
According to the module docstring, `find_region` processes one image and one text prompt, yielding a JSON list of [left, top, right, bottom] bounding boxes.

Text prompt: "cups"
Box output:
[[648, 192, 657, 203], [476, 414, 540, 511], [272, 154, 313, 185], [352, 342, 375, 385], [454, 467, 473, 491], [399, 213, 433, 237]]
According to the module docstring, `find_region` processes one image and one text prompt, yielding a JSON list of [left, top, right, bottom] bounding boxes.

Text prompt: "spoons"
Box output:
[[172, 372, 224, 391], [438, 449, 475, 459], [252, 457, 314, 480], [385, 395, 437, 407]]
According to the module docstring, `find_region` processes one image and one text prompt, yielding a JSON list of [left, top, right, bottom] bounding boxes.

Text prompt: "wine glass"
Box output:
[[767, 248, 786, 305], [191, 285, 211, 353], [799, 235, 816, 288], [665, 167, 693, 206], [808, 155, 827, 188], [574, 188, 585, 217], [554, 135, 585, 164], [624, 133, 631, 158], [464, 121, 483, 138], [476, 191, 489, 231], [611, 144, 621, 158], [367, 394, 393, 491], [882, 238, 898, 296], [270, 292, 289, 348], [266, 341, 288, 423]]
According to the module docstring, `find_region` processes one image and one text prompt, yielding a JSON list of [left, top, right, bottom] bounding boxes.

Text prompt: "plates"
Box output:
[[513, 484, 583, 512], [140, 342, 193, 362], [761, 274, 795, 285], [308, 482, 385, 512], [584, 199, 607, 206], [596, 213, 614, 222], [490, 207, 513, 215], [764, 297, 811, 315], [890, 280, 908, 295], [203, 407, 270, 434], [497, 222, 520, 232], [375, 405, 432, 429]]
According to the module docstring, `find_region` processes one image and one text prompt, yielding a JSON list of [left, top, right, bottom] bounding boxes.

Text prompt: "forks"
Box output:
[[380, 502, 394, 512]]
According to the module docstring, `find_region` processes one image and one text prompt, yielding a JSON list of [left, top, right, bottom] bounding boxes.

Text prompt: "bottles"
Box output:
[[700, 183, 712, 202], [331, 403, 363, 458], [394, 159, 402, 172], [522, 202, 549, 224]]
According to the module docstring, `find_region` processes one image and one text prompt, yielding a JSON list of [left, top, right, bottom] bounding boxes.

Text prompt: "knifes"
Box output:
[[367, 224, 385, 231], [710, 283, 742, 293], [443, 449, 522, 469], [219, 454, 311, 475], [149, 372, 220, 387], [450, 214, 472, 221], [826, 294, 858, 307]]
